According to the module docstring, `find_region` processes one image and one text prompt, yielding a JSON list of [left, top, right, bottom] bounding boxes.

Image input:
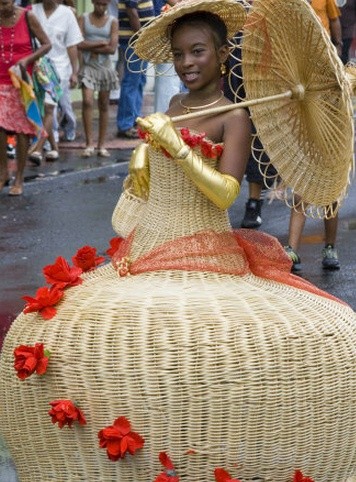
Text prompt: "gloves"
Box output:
[[137, 111, 240, 209], [127, 144, 149, 199]]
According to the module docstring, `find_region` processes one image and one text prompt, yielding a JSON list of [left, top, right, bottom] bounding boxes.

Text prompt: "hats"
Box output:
[[125, 1, 249, 76]]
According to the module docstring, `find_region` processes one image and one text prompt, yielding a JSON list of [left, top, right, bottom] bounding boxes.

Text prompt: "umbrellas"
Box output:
[[141, 0, 355, 220]]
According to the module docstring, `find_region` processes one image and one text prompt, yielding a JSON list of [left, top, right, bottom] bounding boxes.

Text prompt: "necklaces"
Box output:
[[178, 90, 225, 111]]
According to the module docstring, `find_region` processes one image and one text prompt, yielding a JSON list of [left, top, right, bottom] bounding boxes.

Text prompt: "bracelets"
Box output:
[[333, 40, 343, 48], [174, 143, 191, 161]]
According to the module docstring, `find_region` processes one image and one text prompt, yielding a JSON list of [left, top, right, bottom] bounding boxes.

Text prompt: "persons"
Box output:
[[225, 30, 275, 229], [0, 0, 53, 197], [0, 0, 356, 482], [76, 1, 120, 159], [153, 1, 182, 115], [56, 80, 77, 142], [303, 0, 344, 60], [115, 0, 154, 140], [23, 0, 84, 161], [280, 1, 355, 271]]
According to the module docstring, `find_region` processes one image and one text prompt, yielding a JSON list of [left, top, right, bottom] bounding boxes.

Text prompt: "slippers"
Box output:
[[46, 150, 59, 160], [28, 152, 44, 167]]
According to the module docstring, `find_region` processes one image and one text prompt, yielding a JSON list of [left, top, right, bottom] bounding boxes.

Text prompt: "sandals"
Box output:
[[82, 148, 94, 157], [97, 148, 111, 157]]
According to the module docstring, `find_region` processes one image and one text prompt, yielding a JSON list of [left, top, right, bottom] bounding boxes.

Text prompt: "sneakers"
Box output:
[[321, 246, 339, 268], [118, 128, 139, 139], [242, 206, 263, 228], [283, 246, 301, 273]]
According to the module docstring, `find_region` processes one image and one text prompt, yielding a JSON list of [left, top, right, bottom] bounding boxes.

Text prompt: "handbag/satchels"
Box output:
[[35, 56, 65, 119], [111, 188, 145, 240]]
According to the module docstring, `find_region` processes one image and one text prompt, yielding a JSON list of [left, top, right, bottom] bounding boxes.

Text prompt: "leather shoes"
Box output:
[[0, 177, 11, 190], [9, 184, 22, 196]]
